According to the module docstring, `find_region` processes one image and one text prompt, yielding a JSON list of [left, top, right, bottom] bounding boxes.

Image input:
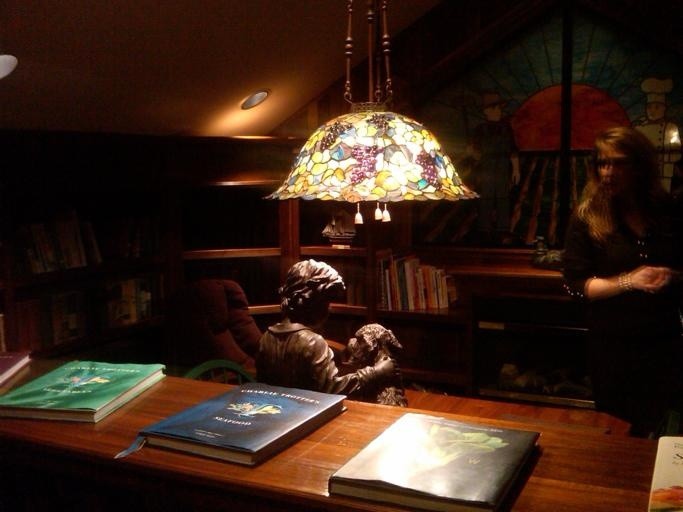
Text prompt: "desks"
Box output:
[[384, 262, 605, 391]]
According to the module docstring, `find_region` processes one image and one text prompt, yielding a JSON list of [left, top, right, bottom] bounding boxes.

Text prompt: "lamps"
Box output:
[[262, 0, 483, 225]]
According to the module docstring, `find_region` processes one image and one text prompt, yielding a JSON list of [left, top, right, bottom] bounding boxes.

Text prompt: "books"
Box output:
[[647, 436, 683, 512], [0, 351, 166, 424], [375, 250, 458, 310], [327, 412, 540, 512], [1, 238, 103, 275], [119, 279, 153, 326], [137, 381, 347, 467]]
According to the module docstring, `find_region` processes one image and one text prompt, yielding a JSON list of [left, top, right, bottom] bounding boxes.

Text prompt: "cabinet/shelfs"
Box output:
[[289, 147, 371, 390], [5, 133, 176, 358], [173, 136, 285, 373]]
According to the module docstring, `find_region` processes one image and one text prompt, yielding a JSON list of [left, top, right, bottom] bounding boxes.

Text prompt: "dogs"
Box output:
[[346, 321, 409, 406]]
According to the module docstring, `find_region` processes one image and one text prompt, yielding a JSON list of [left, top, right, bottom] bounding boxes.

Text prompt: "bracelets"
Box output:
[[618, 273, 632, 291]]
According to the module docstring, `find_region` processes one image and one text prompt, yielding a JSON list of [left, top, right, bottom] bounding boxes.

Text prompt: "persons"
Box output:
[[255, 260, 401, 396], [560, 126, 683, 301], [632, 102, 682, 192], [465, 93, 521, 245]]
